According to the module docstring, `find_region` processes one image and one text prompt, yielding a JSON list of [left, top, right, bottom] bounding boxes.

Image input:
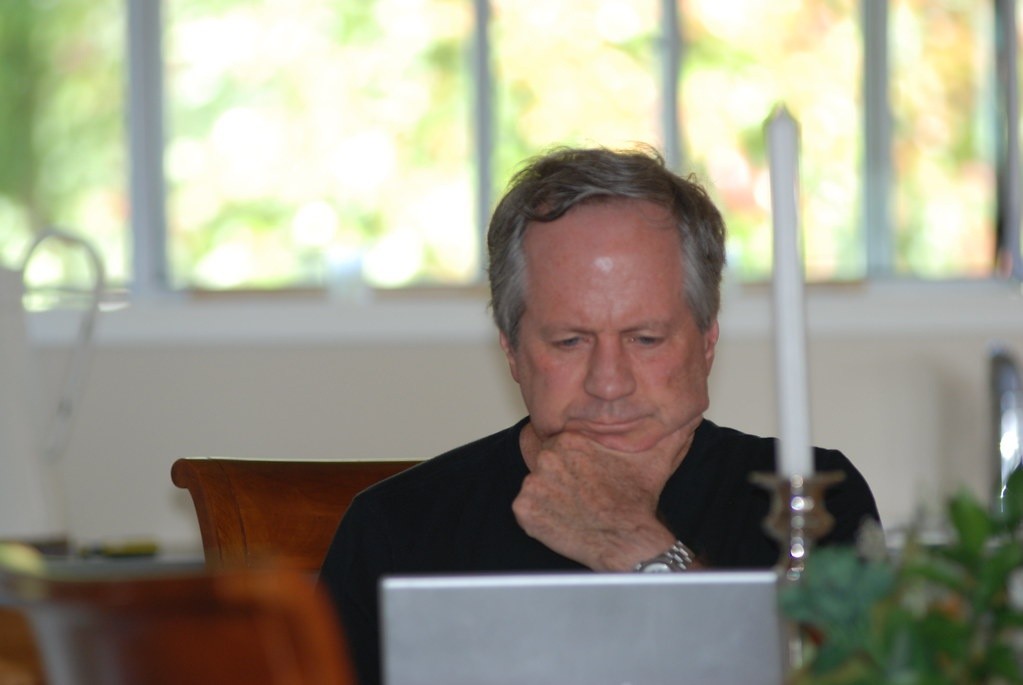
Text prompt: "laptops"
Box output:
[[378, 571, 786, 685]]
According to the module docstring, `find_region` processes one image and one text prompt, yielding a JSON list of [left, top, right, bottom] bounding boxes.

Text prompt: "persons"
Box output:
[[308, 147, 887, 685]]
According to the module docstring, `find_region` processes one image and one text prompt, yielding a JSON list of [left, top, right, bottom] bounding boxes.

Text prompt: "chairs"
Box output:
[[0, 457, 426, 685]]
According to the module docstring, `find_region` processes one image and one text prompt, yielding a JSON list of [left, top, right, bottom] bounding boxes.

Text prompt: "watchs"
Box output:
[[635, 541, 694, 574]]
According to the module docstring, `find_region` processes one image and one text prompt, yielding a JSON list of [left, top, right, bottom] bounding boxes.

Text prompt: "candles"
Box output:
[[769, 104, 815, 477]]
